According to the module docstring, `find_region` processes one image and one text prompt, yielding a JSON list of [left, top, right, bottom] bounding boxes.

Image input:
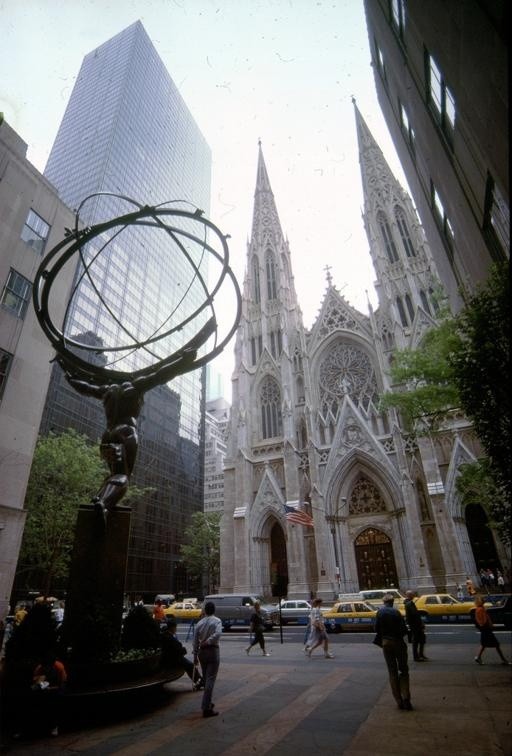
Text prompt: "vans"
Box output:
[[201, 593, 279, 635], [357, 587, 420, 608]]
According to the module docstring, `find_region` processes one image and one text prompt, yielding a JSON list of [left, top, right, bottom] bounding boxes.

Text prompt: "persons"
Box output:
[[475, 595, 509, 665], [32, 657, 70, 736], [373, 595, 414, 708], [13, 604, 28, 627], [456, 568, 511, 601], [303, 599, 333, 660], [244, 600, 270, 656], [134, 599, 222, 718], [404, 590, 429, 662]]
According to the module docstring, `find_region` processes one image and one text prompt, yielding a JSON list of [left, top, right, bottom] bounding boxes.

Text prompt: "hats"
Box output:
[[382, 593, 394, 602]]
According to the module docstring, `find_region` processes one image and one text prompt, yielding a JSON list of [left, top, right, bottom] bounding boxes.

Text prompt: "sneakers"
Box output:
[[473, 655, 486, 665], [200, 701, 218, 718]]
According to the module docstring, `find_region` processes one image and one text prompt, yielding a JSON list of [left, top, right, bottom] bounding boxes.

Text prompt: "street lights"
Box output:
[[302, 497, 347, 595]]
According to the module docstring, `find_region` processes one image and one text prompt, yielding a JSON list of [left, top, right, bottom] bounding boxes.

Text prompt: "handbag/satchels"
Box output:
[[479, 624, 490, 631], [407, 630, 425, 643]]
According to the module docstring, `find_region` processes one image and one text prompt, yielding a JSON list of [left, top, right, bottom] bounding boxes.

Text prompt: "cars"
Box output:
[[321, 603, 379, 634], [269, 599, 332, 625], [51, 599, 65, 624], [401, 593, 492, 620], [469, 596, 511, 633], [142, 593, 203, 624]]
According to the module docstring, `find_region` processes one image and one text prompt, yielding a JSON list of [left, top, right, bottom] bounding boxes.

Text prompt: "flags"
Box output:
[[284, 505, 313, 526]]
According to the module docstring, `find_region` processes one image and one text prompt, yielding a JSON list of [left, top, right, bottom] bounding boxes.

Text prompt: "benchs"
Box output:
[[0, 645, 188, 733]]
